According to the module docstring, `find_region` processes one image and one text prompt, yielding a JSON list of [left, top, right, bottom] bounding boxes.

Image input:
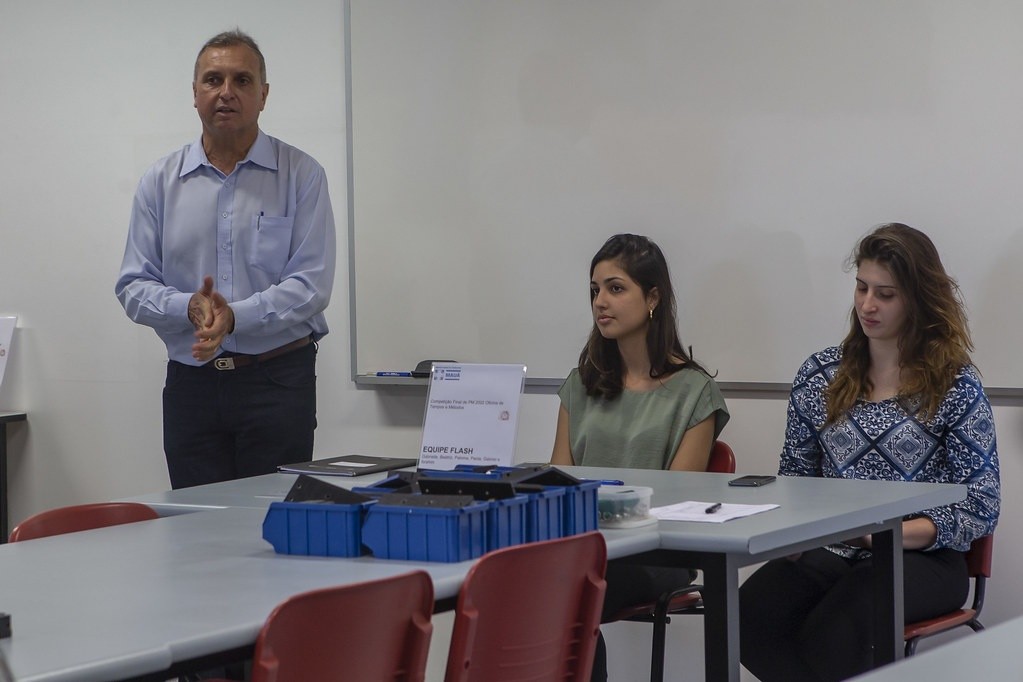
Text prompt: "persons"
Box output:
[[739, 222, 1001, 682], [548, 233, 731, 682], [114, 31, 337, 490]]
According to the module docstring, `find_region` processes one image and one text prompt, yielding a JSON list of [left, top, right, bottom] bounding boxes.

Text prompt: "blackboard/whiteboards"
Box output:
[[343, 1, 1023, 403]]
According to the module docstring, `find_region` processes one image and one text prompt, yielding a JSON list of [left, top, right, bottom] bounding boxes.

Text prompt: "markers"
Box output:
[[375, 372, 411, 377]]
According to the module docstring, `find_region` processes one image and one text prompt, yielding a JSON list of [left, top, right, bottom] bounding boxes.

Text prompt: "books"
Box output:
[[276, 454, 420, 477]]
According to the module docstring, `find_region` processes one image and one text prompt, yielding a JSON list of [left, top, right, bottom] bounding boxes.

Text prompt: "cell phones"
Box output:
[[728, 476, 777, 486]]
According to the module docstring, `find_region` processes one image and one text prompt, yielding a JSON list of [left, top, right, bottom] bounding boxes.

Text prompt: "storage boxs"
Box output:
[[260, 465, 604, 564]]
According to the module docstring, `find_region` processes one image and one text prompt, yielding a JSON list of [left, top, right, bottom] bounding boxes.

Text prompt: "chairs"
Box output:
[[251, 569, 435, 682], [600, 438, 735, 682], [444, 531, 608, 682], [7, 501, 161, 545], [896, 530, 995, 660]]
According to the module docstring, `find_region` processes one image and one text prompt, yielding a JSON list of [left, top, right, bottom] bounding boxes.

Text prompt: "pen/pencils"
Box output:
[[598, 479, 624, 486], [257, 211, 264, 230], [704, 502, 722, 514]]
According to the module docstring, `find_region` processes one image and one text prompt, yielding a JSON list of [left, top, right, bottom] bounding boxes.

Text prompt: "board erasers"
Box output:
[[410, 360, 459, 377]]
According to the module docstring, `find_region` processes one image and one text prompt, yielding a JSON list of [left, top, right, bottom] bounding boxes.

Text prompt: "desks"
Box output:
[[0, 456, 970, 682]]
